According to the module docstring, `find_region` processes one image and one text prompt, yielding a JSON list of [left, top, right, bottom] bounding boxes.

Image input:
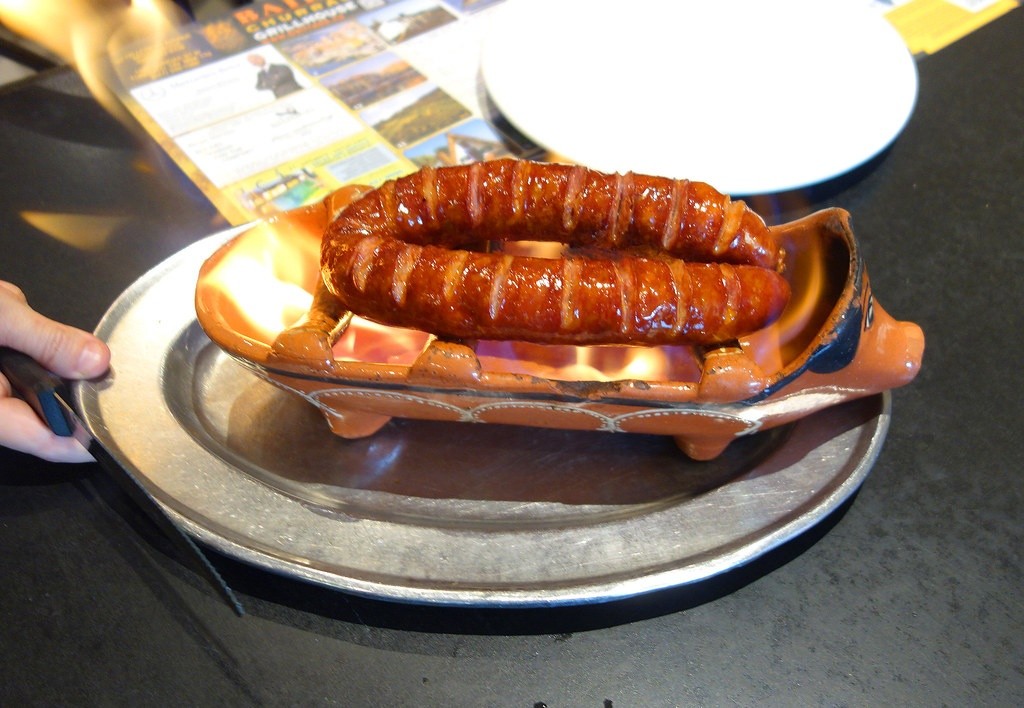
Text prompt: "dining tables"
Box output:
[[0, 0, 1024, 708]]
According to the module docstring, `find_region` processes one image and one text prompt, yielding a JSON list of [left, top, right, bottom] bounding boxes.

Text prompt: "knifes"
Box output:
[[0, 345, 246, 617]]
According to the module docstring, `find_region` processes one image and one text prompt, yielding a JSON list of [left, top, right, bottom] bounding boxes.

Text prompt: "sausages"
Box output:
[[319, 158, 791, 346]]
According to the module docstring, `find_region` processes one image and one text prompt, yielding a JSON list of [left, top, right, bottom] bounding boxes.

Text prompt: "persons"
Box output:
[[0, 281, 111, 463], [247, 53, 302, 99]]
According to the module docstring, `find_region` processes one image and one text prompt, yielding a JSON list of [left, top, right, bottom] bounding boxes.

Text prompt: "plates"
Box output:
[[476, 0, 921, 199], [70, 218, 892, 611]]
